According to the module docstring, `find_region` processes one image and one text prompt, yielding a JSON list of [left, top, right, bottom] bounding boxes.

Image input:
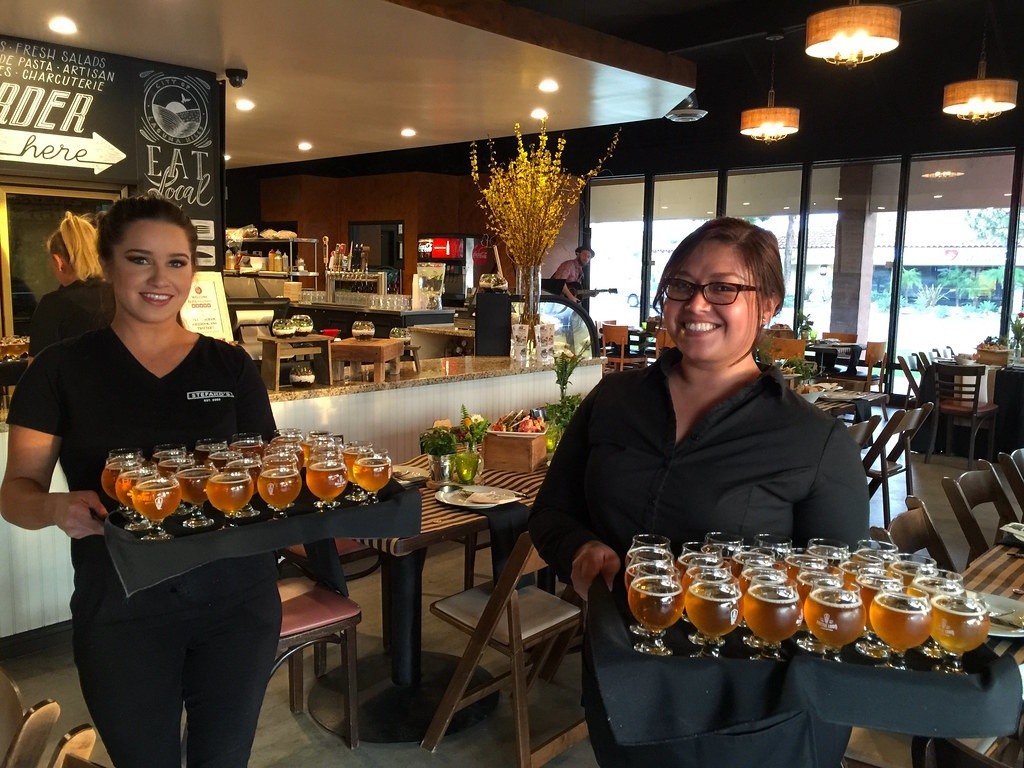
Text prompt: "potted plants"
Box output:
[[784, 353, 814, 387], [418, 425, 458, 483]]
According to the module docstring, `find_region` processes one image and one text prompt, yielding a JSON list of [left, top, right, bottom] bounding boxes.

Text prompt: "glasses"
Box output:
[[662, 278, 763, 305]]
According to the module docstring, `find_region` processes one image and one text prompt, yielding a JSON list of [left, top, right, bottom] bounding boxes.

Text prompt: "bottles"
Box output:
[[274, 314, 314, 339], [329, 242, 370, 270], [352, 321, 375, 342], [389, 327, 412, 345], [332, 338, 344, 382], [480, 273, 508, 294], [225, 247, 306, 271], [288, 365, 315, 388]]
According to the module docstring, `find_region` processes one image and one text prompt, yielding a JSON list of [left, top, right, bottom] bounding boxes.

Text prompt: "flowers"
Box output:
[[1007, 312, 1024, 341], [450, 403, 489, 445], [551, 338, 591, 403], [795, 308, 814, 336], [469, 110, 621, 264]]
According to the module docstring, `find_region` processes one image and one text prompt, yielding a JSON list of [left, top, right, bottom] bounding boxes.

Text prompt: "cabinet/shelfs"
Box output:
[[224, 237, 320, 292]]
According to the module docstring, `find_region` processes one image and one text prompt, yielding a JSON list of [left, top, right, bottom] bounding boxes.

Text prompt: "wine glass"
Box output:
[[0, 334, 30, 363], [622, 532, 990, 676], [101, 426, 392, 541]]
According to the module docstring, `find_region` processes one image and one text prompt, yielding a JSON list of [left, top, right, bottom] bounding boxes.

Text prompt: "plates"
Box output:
[[936, 357, 954, 361], [783, 374, 803, 379], [1012, 367, 1024, 371], [964, 590, 1024, 638], [434, 485, 526, 509], [825, 386, 843, 392], [390, 464, 429, 485], [486, 422, 549, 439]]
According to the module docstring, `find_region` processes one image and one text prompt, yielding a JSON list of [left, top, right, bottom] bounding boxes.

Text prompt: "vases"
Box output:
[[1013, 340, 1022, 364], [455, 441, 480, 482], [976, 348, 1013, 368], [516, 264, 541, 357], [801, 330, 808, 340], [544, 420, 559, 450]]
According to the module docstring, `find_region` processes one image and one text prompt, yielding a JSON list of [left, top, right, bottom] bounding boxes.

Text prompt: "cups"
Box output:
[[546, 429, 558, 453], [641, 322, 647, 330], [298, 290, 412, 311], [810, 331, 817, 342], [511, 323, 555, 362], [454, 453, 479, 484]]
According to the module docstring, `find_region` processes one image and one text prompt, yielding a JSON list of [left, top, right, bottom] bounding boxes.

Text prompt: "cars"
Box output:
[[625, 281, 663, 310]]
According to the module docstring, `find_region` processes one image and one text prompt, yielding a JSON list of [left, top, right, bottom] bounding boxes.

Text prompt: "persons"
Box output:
[[528, 218, 872, 768], [548, 246, 595, 308], [28, 210, 115, 370], [1, 200, 284, 768]]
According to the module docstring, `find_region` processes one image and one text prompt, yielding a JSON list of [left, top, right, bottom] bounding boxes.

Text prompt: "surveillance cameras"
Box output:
[[225, 69, 247, 89]]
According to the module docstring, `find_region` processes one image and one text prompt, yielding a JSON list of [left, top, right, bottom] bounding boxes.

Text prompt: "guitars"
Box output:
[[564, 282, 618, 303]]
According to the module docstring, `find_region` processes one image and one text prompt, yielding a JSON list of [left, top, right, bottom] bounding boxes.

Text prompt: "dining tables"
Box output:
[[805, 341, 867, 377], [812, 388, 890, 450], [910, 359, 1024, 463], [599, 325, 656, 367], [306, 446, 551, 745], [954, 541, 1024, 667]]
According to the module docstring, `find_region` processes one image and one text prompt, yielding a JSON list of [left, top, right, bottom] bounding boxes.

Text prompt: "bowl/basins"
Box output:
[[999, 522, 1024, 542], [825, 338, 840, 343], [818, 383, 838, 387], [249, 257, 268, 269], [954, 356, 976, 365], [797, 389, 825, 403]]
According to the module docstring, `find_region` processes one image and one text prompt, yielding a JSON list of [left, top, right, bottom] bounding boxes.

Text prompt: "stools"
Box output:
[[256, 334, 334, 393], [331, 337, 405, 384], [386, 345, 421, 374]]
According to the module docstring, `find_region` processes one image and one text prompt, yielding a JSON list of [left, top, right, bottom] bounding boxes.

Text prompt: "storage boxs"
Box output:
[[483, 434, 547, 473]]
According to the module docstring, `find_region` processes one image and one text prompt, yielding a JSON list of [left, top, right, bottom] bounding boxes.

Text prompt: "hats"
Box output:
[[575, 246, 595, 258]]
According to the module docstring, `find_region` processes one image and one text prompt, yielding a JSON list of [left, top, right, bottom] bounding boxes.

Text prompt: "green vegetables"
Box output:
[[546, 393, 582, 429], [782, 354, 814, 380]]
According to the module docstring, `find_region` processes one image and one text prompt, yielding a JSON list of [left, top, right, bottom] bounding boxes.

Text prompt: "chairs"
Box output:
[[600, 318, 1024, 573], [419, 531, 590, 768], [911, 735, 1014, 768], [840, 725, 1002, 768], [268, 574, 362, 751], [279, 434, 377, 680]]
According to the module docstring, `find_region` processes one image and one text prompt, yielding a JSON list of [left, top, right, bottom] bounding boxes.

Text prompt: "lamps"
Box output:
[[739, 26, 799, 145], [805, 0, 902, 71], [942, 9, 1019, 125]]
